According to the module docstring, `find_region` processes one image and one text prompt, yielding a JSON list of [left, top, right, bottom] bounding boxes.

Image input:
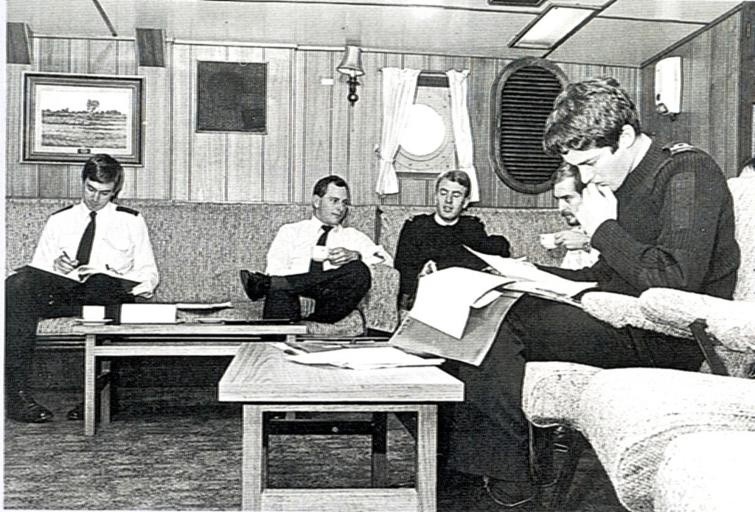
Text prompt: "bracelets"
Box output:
[[354, 250, 362, 261], [580, 235, 593, 253]]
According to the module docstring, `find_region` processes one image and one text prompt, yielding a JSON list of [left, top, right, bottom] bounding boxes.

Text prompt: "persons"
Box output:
[[538, 163, 602, 269], [4, 154, 160, 422], [239, 174, 380, 342], [393, 170, 511, 311], [442, 73, 741, 512]]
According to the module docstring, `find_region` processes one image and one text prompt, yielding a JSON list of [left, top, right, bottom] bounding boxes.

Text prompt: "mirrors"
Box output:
[[400, 101, 449, 159]]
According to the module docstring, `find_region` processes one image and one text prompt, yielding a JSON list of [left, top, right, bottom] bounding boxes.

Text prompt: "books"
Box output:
[[411, 265, 516, 336], [12, 259, 143, 290]]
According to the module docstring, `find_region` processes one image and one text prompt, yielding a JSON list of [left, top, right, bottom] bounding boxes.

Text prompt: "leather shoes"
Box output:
[[239, 270, 271, 302], [7, 389, 54, 423], [67, 392, 120, 420]]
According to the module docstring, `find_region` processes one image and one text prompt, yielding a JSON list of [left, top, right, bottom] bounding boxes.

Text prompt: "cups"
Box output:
[[309, 246, 334, 263], [83, 305, 105, 319], [538, 232, 557, 249]]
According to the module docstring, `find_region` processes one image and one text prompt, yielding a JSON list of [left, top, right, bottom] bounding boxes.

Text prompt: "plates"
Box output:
[[74, 318, 113, 326]]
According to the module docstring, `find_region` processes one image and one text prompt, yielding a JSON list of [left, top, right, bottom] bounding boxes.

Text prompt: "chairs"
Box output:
[[522, 176, 751, 512], [548, 270, 751, 510]]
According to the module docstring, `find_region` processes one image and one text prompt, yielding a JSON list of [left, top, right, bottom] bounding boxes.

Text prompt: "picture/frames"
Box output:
[[22, 71, 148, 167]]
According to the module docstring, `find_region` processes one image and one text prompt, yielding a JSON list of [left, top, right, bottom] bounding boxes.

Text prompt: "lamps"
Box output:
[[336, 43, 366, 105]]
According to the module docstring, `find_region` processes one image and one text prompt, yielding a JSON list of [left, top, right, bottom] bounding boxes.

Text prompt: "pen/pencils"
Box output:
[[62, 250, 70, 260], [481, 256, 528, 271]]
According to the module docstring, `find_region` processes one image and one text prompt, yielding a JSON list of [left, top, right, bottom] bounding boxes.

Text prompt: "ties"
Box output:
[[74, 210, 97, 281], [309, 224, 333, 273]]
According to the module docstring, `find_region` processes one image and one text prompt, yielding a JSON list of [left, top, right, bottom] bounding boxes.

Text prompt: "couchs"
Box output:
[[380, 203, 569, 330], [7, 197, 400, 392]]
